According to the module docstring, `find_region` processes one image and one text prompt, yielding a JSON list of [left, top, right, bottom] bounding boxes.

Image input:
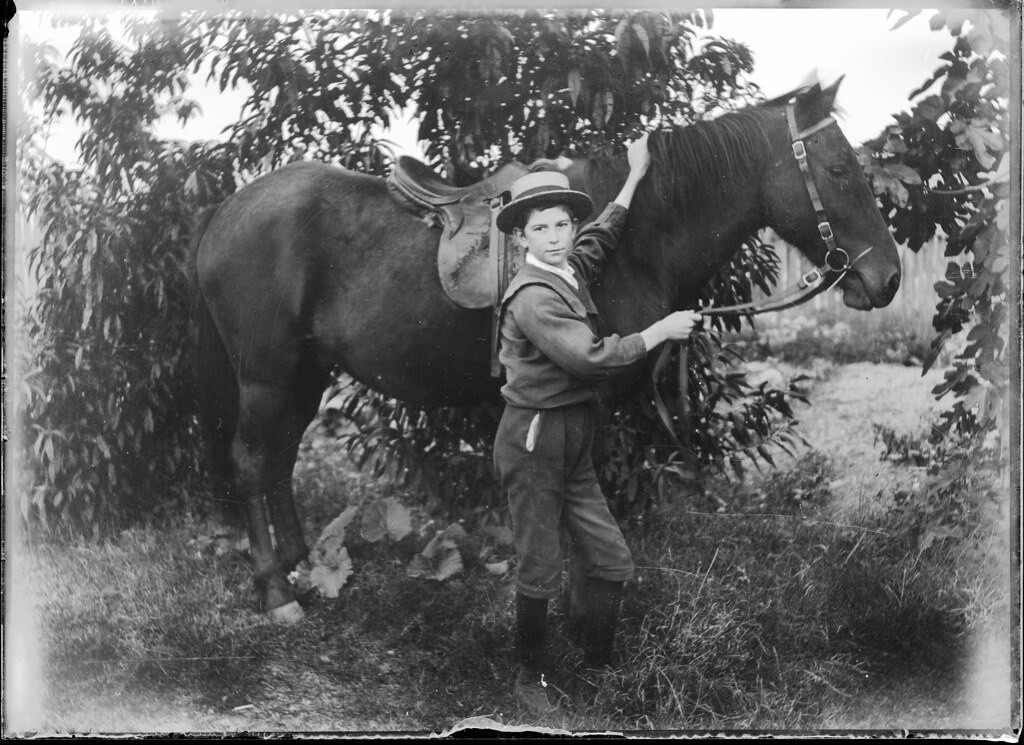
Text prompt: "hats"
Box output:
[[497, 171, 593, 234]]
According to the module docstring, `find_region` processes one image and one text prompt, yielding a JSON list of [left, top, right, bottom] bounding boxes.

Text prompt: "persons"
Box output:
[[492, 132, 702, 720]]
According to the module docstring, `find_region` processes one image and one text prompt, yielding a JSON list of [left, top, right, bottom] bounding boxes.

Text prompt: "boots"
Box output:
[[581, 576, 624, 704], [514, 592, 560, 718]]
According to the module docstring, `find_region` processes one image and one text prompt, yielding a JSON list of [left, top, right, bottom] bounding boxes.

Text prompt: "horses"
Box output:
[[188, 72, 904, 630]]
